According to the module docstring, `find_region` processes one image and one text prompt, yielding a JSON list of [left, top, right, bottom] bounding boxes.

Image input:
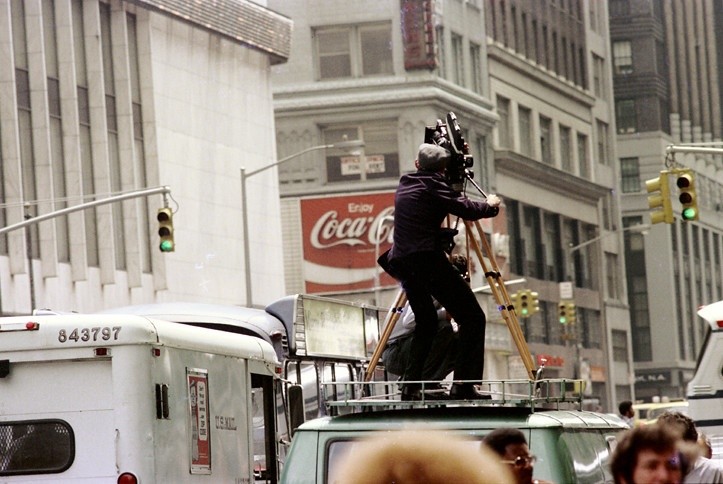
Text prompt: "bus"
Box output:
[[0, 315, 306, 484]]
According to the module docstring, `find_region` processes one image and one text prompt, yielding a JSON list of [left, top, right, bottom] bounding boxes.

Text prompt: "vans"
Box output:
[[279, 376, 632, 484], [687, 299, 723, 441], [632, 401, 690, 430]]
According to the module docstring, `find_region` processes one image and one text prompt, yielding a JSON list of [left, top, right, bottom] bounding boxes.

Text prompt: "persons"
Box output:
[[337, 408, 723, 484], [619, 400, 634, 428], [377, 142, 501, 400]]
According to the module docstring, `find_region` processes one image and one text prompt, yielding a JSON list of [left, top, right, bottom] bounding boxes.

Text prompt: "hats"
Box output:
[[418, 144, 452, 168]]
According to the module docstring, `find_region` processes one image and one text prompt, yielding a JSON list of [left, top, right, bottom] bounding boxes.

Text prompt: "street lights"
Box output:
[[565, 222, 652, 379], [240, 133, 365, 306]]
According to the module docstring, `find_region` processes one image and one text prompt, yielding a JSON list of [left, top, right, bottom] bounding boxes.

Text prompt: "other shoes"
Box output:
[[421, 383, 448, 400], [401, 384, 420, 400], [450, 384, 492, 400]]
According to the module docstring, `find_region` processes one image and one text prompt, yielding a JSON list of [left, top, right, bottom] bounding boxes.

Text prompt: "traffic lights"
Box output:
[[677, 172, 700, 221], [558, 302, 566, 323], [527, 290, 539, 315], [157, 207, 175, 252], [511, 290, 521, 317], [567, 304, 577, 325], [645, 173, 673, 224], [517, 289, 534, 318]]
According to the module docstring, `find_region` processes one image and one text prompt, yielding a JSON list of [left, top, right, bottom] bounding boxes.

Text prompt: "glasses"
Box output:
[[502, 455, 536, 469]]
